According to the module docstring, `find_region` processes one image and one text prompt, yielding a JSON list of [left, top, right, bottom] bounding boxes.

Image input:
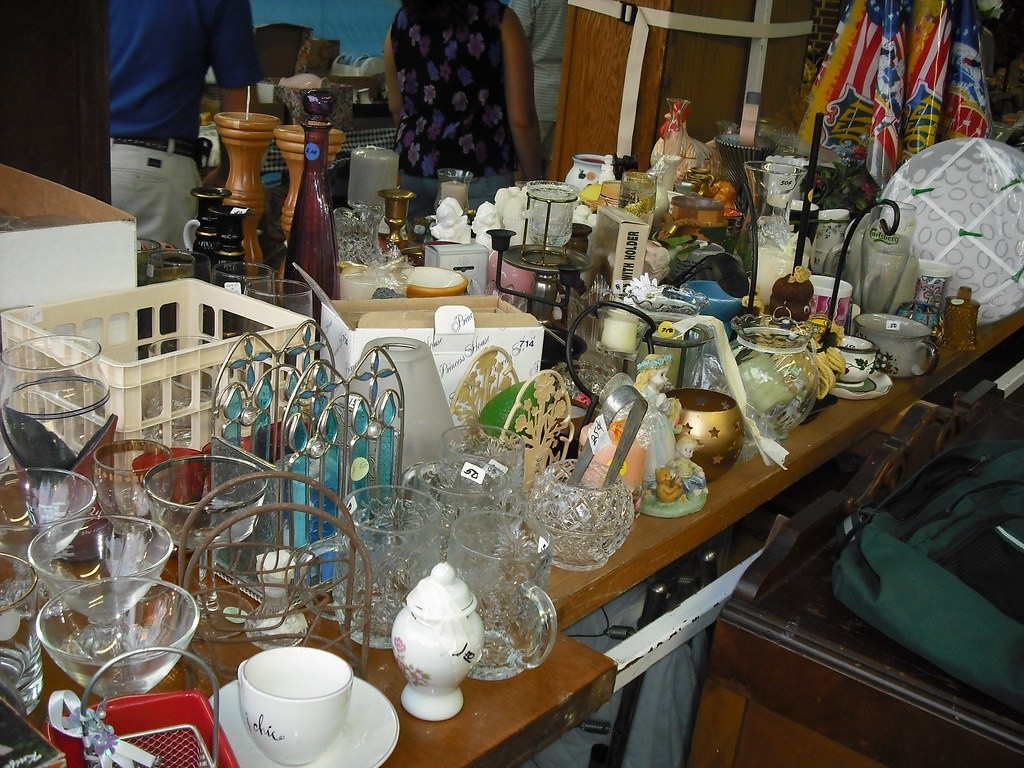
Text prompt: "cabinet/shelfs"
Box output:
[[687, 378, 1024, 768]]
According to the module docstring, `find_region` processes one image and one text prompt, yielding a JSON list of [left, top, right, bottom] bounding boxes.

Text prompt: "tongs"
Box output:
[[565, 385, 648, 489]]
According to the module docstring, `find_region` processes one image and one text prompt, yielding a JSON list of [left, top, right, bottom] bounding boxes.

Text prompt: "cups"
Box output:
[[36, 577, 200, 699], [442, 424, 525, 497], [0, 552, 44, 716], [620, 171, 656, 237], [142, 333, 222, 445], [333, 205, 468, 299], [294, 485, 441, 649], [448, 511, 558, 679], [681, 280, 743, 340], [412, 456, 528, 591], [0, 334, 110, 620], [93, 421, 309, 538], [528, 459, 636, 571], [238, 647, 354, 766], [835, 335, 876, 387], [136, 237, 313, 383], [767, 200, 954, 347], [27, 515, 174, 657], [565, 153, 605, 190], [854, 313, 939, 377], [530, 181, 576, 246]]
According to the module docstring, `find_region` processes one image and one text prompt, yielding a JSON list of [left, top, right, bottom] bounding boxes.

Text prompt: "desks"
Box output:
[[0, 474, 619, 768], [262, 127, 397, 171], [548, 311, 1024, 632]]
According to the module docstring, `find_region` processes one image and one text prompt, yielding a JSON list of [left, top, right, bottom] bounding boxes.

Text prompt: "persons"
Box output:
[[385, 0, 543, 217], [508, 0, 569, 180], [109, 0, 261, 248]]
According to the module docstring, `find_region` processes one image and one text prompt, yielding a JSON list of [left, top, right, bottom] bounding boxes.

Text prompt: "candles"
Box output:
[[434, 167, 474, 214]]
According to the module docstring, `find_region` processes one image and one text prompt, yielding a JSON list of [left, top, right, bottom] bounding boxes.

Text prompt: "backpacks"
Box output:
[[836, 440, 1023, 713]]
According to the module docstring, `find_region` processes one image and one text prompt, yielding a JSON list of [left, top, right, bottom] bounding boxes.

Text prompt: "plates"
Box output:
[[208, 676, 400, 768], [829, 369, 893, 400]]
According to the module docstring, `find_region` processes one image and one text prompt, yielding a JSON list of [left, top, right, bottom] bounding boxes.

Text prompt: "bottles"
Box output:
[[278, 74, 329, 88], [245, 586, 308, 650], [284, 91, 340, 380], [743, 160, 808, 305]]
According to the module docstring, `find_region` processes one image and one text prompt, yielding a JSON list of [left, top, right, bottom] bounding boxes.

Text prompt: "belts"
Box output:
[[114, 133, 198, 158]]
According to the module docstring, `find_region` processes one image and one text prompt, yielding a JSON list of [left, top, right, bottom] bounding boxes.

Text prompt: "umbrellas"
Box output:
[[794, 0, 992, 190]]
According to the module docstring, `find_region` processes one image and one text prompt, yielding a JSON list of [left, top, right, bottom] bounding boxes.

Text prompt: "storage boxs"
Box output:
[[294, 37, 341, 76], [587, 202, 650, 309], [325, 71, 386, 100], [289, 262, 548, 457], [0, 164, 146, 450], [253, 23, 314, 78], [0, 279, 318, 484]]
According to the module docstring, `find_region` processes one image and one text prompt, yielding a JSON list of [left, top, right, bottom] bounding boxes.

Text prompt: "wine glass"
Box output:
[[144, 455, 268, 641]]
